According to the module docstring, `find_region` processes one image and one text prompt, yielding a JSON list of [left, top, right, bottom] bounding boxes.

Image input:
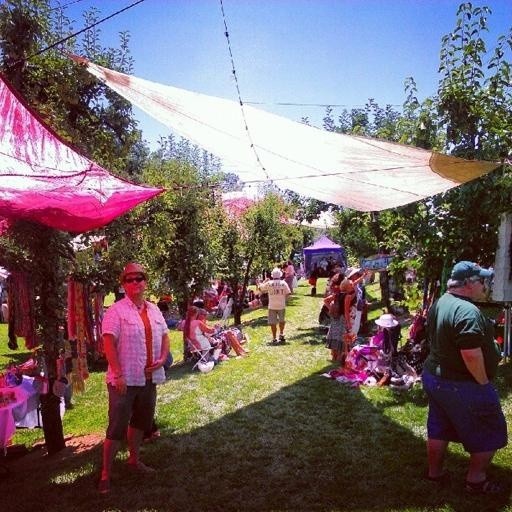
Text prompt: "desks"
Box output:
[[1, 394, 44, 456]]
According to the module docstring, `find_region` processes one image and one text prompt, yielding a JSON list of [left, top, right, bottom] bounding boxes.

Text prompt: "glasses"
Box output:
[[476, 277, 485, 284], [124, 277, 145, 283]]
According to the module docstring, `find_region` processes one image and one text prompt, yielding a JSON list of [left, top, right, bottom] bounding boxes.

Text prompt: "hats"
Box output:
[[375, 314, 398, 328], [344, 267, 362, 279], [271, 268, 283, 278], [451, 261, 495, 280]]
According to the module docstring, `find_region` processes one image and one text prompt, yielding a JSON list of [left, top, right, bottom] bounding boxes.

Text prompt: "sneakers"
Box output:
[[126, 460, 154, 474], [98, 479, 111, 494], [273, 334, 284, 344]]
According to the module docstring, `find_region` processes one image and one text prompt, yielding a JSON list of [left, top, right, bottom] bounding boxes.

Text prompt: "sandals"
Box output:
[[466, 478, 502, 495]]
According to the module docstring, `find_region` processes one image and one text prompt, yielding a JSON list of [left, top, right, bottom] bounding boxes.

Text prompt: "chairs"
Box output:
[[184, 336, 214, 371]]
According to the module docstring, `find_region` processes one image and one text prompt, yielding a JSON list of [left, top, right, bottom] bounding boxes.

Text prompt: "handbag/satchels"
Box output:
[[329, 300, 340, 318]]
